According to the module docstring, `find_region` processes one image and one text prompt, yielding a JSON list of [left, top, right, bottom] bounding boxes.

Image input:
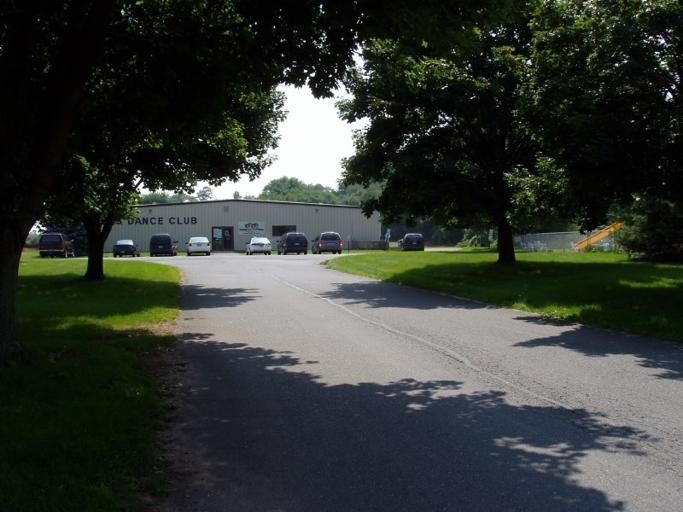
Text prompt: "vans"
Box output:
[[276, 232, 308, 255], [150, 233, 178, 256]]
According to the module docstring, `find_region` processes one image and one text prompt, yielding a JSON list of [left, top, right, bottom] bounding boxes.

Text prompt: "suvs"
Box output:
[[39, 232, 75, 258]]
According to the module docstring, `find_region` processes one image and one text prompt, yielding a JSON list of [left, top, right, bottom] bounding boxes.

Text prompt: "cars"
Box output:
[[246, 237, 272, 255], [112, 239, 140, 257], [311, 232, 344, 254], [185, 237, 211, 256], [398, 232, 424, 251]]
